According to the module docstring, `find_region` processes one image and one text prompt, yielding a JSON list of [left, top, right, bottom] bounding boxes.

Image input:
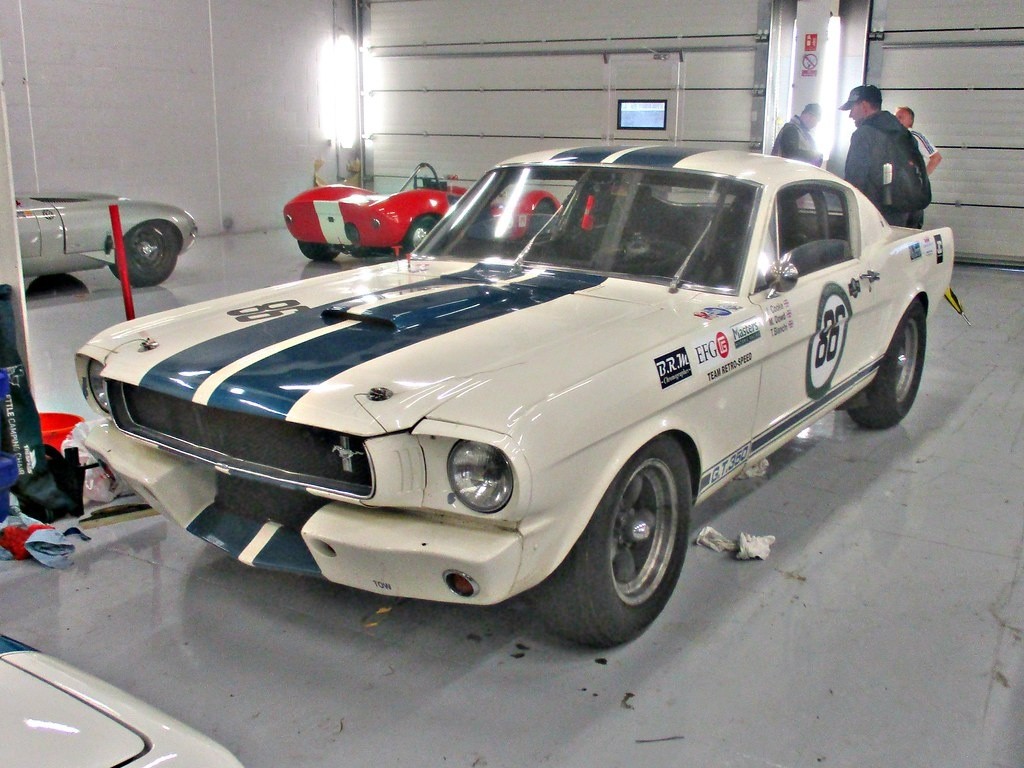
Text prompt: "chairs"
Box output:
[[413, 163, 441, 190]]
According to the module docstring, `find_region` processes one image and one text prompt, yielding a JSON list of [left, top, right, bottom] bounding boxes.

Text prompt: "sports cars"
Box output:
[[282, 159, 566, 263]]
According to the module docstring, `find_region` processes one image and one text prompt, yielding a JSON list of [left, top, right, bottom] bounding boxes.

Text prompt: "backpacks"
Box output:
[[860, 119, 933, 213]]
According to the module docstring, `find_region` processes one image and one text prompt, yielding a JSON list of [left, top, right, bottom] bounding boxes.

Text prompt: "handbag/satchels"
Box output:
[[11, 442, 86, 523], [60, 420, 132, 503]]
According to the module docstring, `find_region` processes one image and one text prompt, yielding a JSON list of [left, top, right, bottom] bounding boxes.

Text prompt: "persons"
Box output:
[[839, 85, 932, 229], [771, 103, 822, 167], [894, 107, 942, 177]]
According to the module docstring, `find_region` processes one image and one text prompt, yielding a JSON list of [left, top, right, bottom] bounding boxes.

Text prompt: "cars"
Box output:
[[14, 192, 201, 289], [72, 143, 955, 650]]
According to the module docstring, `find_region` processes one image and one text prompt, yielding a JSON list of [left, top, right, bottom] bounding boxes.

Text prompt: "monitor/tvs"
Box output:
[[618, 99, 667, 131]]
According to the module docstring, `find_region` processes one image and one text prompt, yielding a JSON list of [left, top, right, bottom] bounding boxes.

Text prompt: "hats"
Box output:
[[838, 85, 882, 110]]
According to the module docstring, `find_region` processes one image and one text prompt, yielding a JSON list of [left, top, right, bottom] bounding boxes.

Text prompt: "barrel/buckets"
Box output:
[[38, 412, 85, 452]]
